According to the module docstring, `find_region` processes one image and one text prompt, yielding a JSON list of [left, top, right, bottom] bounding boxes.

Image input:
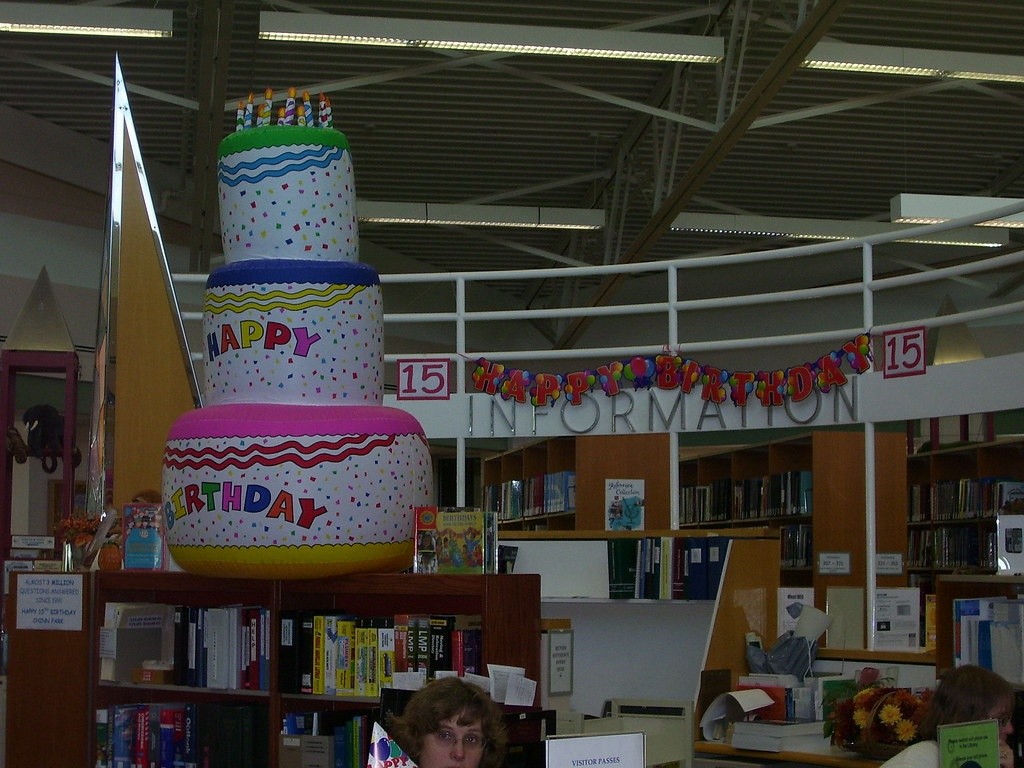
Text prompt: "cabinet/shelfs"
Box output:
[[480, 434, 670, 528], [91, 570, 541, 768], [679, 430, 909, 649], [908, 436, 1024, 681]]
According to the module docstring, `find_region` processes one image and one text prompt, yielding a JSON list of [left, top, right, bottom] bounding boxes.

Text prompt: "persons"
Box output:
[[878, 663, 1017, 768], [401, 679, 508, 768]]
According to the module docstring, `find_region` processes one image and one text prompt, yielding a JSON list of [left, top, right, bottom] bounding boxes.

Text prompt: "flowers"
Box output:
[[819, 666, 933, 749]]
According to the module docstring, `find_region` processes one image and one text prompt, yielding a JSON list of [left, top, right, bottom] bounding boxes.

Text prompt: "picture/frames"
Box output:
[[825, 586, 866, 646], [548, 628, 573, 695]]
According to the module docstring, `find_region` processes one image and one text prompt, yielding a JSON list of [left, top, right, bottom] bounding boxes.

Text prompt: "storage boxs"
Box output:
[[278, 733, 334, 768], [132, 667, 172, 683]]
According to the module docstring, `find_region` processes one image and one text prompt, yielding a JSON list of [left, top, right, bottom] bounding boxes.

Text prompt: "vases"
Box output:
[[841, 740, 906, 760]]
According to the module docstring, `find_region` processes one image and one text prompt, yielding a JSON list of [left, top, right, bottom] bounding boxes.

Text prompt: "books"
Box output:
[[725, 670, 857, 753], [411, 506, 518, 573], [607, 538, 728, 601], [936, 719, 1001, 768], [908, 526, 997, 569], [784, 524, 812, 568], [907, 477, 1024, 523], [953, 596, 1024, 688], [99, 603, 481, 697], [484, 472, 577, 521], [679, 469, 812, 524], [94, 700, 368, 768]]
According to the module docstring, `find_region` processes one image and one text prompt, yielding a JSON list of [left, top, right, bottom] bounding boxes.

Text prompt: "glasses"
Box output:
[[433, 730, 489, 752], [984, 711, 1013, 728]]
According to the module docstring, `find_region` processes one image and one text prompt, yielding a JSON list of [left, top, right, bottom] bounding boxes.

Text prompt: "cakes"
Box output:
[[158, 126, 437, 581]]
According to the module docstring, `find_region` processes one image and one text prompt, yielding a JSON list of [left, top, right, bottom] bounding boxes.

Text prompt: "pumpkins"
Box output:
[[98, 541, 120, 570]]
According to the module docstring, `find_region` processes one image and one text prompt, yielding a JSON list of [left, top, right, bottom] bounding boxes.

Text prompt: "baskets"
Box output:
[[842, 692, 907, 761]]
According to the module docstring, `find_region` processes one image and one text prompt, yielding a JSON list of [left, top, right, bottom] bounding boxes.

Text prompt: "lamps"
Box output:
[[666, 64, 1010, 246], [801, 41, 1024, 82], [0, 2, 173, 38], [890, 0, 1024, 230], [355, 133, 604, 231], [258, 0, 723, 63]]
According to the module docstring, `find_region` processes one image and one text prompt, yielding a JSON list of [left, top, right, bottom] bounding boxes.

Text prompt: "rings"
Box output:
[[1002, 751, 1008, 759]]
